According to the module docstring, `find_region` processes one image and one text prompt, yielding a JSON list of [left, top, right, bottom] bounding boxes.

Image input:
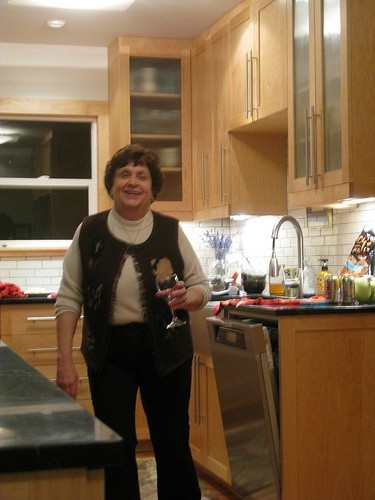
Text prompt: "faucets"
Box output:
[[271, 215, 305, 299]]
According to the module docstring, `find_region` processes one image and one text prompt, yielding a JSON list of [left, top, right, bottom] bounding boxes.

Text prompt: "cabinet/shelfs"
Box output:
[[226, 0, 287, 133], [285, 0, 375, 209], [192, 26, 285, 218], [186, 351, 234, 488], [106, 37, 193, 223], [0, 304, 94, 417]]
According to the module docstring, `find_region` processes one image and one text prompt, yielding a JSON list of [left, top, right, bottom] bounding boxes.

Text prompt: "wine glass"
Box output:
[[156, 273, 186, 329]]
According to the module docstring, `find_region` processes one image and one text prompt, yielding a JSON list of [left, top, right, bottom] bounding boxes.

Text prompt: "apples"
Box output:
[[355, 275, 375, 303]]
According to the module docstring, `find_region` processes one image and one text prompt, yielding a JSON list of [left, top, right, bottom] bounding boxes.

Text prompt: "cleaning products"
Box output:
[[315, 258, 332, 296]]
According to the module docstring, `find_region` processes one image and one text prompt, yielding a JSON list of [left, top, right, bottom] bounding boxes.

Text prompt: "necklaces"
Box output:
[[117, 218, 144, 248]]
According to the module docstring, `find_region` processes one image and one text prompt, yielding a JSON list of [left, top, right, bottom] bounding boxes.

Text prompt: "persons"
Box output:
[[54, 143, 213, 500]]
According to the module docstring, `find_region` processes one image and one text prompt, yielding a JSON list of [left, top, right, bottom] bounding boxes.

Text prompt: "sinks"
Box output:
[[210, 296, 290, 301]]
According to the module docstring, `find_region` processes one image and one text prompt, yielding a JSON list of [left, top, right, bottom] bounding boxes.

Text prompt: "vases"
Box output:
[[215, 258, 228, 277]]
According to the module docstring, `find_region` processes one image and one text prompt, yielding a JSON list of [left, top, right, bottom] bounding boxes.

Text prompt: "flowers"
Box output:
[[199, 229, 239, 260]]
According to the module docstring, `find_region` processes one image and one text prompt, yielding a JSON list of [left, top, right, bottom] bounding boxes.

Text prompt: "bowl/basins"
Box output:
[[241, 271, 267, 295], [160, 146, 179, 167]]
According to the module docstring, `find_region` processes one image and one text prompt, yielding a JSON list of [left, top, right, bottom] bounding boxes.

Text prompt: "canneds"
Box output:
[[324, 273, 356, 306]]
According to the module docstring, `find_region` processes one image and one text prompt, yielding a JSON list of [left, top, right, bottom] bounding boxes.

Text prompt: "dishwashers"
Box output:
[[205, 313, 279, 500]]
